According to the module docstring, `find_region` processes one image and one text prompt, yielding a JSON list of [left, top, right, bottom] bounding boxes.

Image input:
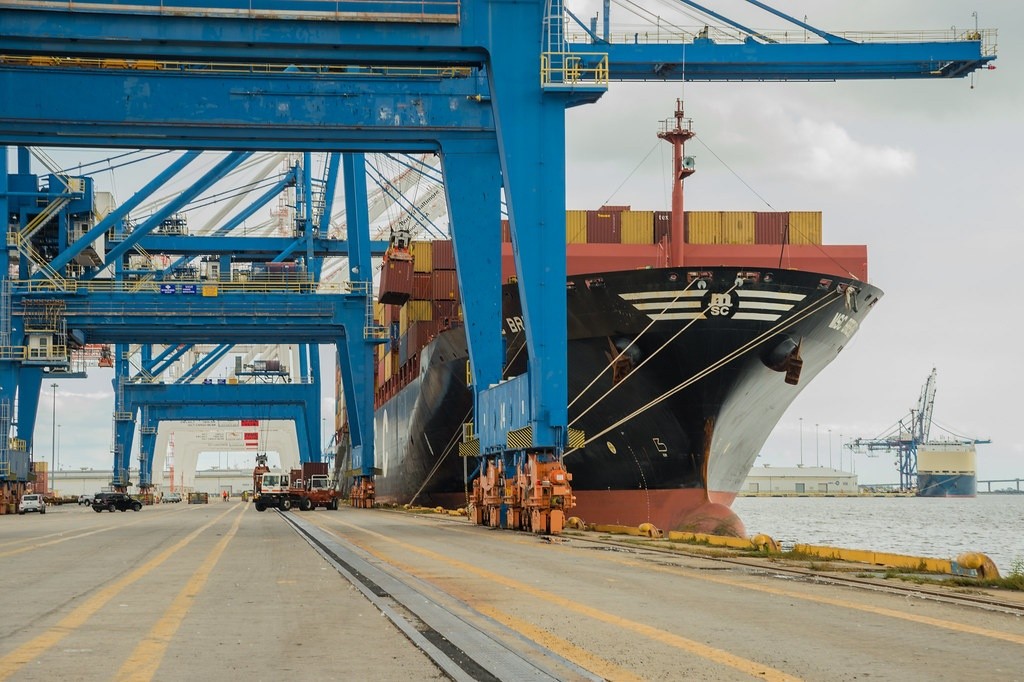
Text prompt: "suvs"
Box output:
[[92, 493, 143, 513]]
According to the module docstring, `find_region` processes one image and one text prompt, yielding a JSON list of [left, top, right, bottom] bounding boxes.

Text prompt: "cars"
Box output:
[[162, 493, 182, 504], [19, 495, 46, 515], [78, 493, 98, 506]]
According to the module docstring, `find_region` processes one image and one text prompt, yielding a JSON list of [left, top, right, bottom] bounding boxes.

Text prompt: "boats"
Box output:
[[329, 95, 885, 539]]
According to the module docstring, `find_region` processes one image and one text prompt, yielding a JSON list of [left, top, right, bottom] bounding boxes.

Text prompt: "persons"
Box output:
[[223, 491, 230, 502]]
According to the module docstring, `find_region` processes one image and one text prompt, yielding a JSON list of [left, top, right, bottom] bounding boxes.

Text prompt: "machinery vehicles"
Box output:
[[256, 473, 336, 511]]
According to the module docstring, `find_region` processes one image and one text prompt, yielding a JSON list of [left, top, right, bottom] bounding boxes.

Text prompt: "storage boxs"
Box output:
[[335, 210, 822, 440]]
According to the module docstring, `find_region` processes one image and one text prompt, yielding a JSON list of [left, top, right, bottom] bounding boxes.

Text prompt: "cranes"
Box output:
[[848, 366, 994, 498]]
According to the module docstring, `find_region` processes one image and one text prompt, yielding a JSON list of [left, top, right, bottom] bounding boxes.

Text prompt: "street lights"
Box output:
[[827, 430, 832, 467], [814, 423, 818, 467], [838, 435, 842, 469], [797, 417, 803, 462], [50, 383, 59, 489]]
